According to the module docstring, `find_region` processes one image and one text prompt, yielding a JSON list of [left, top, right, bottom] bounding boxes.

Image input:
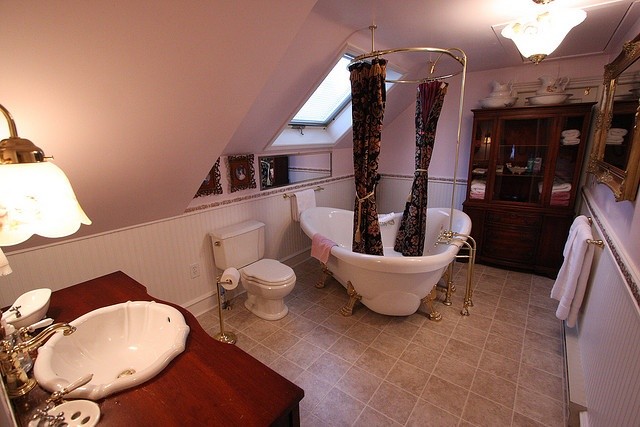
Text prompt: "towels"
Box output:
[[539, 182, 572, 193], [292, 189, 318, 224], [471, 180, 486, 193], [563, 137, 580, 146], [550, 193, 571, 207], [310, 233, 338, 264], [607, 126, 627, 145], [562, 215, 588, 258], [469, 191, 485, 200], [550, 226, 594, 328], [562, 129, 580, 139]]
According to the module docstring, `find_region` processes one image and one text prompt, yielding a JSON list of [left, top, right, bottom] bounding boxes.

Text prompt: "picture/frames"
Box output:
[[193, 157, 224, 198], [224, 154, 257, 194]]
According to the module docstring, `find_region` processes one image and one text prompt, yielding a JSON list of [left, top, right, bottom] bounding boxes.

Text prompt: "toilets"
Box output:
[[209, 220, 296, 320]]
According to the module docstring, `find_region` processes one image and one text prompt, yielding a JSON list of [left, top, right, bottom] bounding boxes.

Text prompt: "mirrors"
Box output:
[[586, 33, 640, 202], [258, 151, 332, 192]]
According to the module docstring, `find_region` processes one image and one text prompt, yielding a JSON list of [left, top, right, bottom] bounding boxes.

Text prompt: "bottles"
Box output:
[[533, 152, 542, 174], [527, 153, 534, 172]]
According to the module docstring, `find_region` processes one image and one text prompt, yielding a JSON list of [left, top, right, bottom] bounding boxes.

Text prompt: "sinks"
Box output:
[[33, 299, 190, 400]]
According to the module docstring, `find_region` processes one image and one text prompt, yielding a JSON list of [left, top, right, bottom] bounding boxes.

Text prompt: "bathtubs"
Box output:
[[299, 207, 477, 322]]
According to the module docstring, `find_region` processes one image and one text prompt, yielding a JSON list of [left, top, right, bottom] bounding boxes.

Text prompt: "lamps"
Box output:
[[0, 98, 93, 250], [501, 1, 587, 65]]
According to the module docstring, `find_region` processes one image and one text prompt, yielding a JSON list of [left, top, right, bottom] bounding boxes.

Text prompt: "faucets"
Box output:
[[0, 321, 77, 401], [0, 305, 22, 321]]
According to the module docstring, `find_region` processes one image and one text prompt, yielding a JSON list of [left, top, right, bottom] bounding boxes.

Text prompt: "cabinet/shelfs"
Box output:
[[456, 203, 486, 262], [482, 207, 543, 274], [468, 101, 598, 208], [535, 207, 577, 279]]
